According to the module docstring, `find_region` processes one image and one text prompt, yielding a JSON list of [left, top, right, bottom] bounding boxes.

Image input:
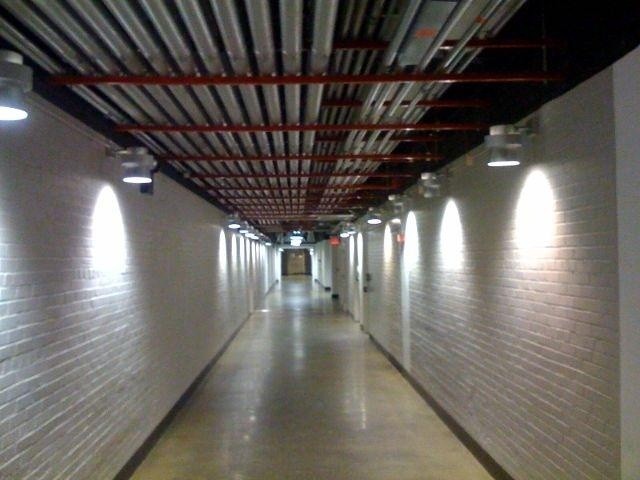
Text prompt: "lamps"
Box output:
[[339, 122, 529, 239], [0, 48, 35, 122], [289, 228, 304, 247], [106, 145, 157, 184], [225, 211, 273, 248]]
